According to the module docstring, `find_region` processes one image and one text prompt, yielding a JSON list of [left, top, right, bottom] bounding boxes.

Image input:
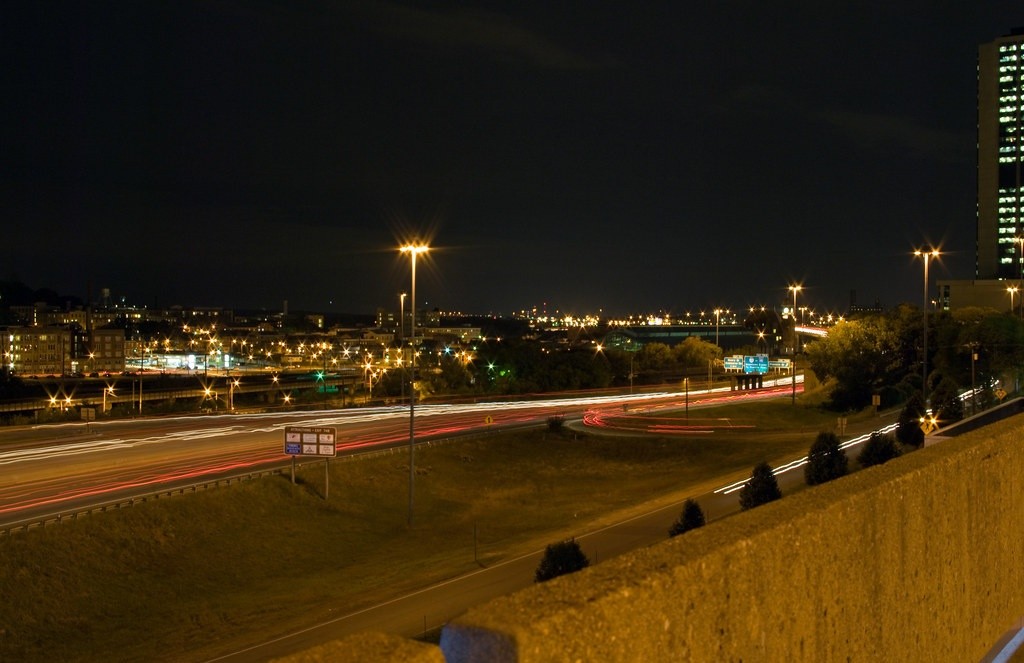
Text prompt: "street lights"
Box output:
[[231, 380, 240, 410], [1014, 237, 1024, 287], [391, 244, 431, 530], [364, 363, 370, 404], [787, 284, 804, 405], [400, 294, 407, 405], [369, 373, 376, 399], [103, 388, 114, 412], [1006, 287, 1019, 314], [912, 248, 942, 405], [713, 309, 723, 347]]
